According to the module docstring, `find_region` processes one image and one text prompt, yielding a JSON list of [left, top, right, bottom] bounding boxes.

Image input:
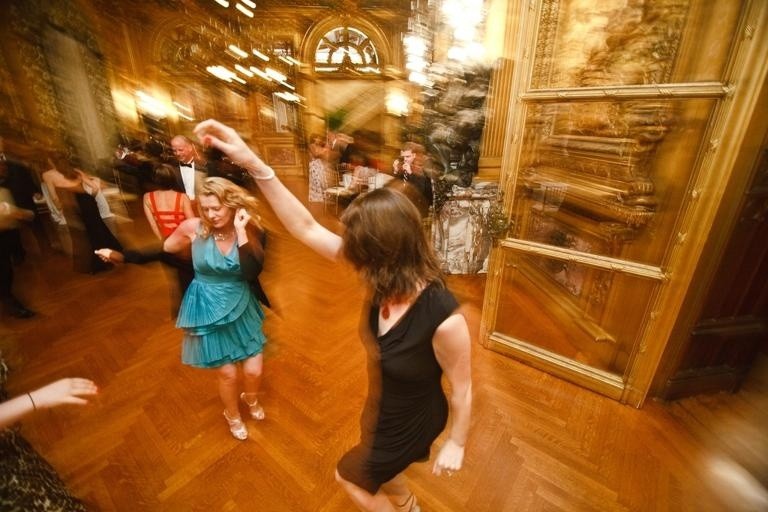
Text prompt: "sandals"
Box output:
[[240, 392, 265, 420], [223, 408, 248, 440]]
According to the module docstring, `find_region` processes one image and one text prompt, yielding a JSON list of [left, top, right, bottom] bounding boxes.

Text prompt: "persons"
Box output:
[[191, 117, 475, 512], [0, 359, 99, 512], [94, 175, 268, 444], [0, 131, 433, 323]]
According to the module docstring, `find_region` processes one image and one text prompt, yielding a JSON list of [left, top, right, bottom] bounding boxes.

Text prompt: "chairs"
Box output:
[[336, 164, 351, 186], [359, 175, 376, 194], [102, 169, 129, 213], [322, 167, 348, 215]]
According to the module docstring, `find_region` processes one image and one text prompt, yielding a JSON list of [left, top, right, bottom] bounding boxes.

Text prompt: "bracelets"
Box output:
[[26, 391, 38, 412]]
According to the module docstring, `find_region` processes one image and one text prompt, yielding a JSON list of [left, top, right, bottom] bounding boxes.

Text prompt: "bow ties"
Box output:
[[181, 161, 192, 168]]
